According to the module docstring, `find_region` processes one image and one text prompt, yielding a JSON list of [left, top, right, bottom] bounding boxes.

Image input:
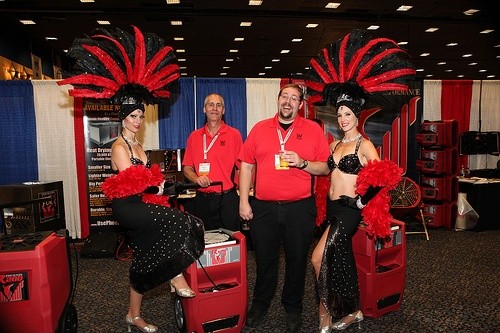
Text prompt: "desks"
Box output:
[[458, 181, 500, 231]]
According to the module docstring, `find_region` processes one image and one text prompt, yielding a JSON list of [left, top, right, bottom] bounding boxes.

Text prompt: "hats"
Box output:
[[57, 23, 182, 120], [294, 30, 417, 118]]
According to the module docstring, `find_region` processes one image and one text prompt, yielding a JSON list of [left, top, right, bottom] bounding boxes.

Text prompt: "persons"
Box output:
[[101, 100, 205, 333], [311, 94, 403, 333], [235, 84, 331, 333], [181, 94, 243, 231]]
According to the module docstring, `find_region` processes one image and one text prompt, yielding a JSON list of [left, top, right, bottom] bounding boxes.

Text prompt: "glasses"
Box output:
[[280, 93, 301, 104]]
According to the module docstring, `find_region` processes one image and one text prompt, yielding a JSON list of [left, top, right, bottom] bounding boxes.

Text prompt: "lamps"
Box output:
[[8, 63, 32, 81]]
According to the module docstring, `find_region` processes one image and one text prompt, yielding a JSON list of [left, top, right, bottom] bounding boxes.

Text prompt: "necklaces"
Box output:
[[123, 135, 139, 146], [342, 135, 361, 142]]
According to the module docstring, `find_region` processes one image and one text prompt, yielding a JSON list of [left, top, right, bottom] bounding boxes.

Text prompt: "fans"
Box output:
[[391, 178, 430, 241]]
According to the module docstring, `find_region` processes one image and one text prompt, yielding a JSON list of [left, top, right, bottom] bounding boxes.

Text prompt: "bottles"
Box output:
[[460, 167, 465, 177], [465, 167, 470, 178]]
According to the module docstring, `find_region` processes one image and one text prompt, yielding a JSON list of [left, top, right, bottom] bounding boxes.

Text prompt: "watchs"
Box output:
[[300, 159, 308, 170]]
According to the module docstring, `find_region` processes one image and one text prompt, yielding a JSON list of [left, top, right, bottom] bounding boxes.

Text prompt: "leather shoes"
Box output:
[[245, 299, 269, 326], [285, 312, 302, 333]]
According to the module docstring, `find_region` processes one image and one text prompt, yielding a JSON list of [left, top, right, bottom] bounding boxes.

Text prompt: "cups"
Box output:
[[279, 150, 289, 167]]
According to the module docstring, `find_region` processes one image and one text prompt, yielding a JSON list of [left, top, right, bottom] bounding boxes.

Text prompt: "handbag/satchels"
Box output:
[[78, 233, 118, 258]]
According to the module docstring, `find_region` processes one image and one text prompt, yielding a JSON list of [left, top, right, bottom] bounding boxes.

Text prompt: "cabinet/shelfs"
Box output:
[[416, 118, 458, 227]]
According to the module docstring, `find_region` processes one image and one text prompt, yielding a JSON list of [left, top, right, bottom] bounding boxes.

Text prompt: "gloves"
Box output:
[[141, 192, 185, 212], [336, 194, 367, 209]]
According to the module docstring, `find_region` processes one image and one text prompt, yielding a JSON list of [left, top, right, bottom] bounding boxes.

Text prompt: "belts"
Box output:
[[198, 187, 236, 197], [255, 194, 314, 204]]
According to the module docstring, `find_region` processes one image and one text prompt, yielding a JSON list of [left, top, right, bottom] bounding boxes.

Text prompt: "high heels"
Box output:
[[169, 273, 197, 297], [318, 312, 332, 333], [332, 310, 365, 330], [125, 312, 158, 333]]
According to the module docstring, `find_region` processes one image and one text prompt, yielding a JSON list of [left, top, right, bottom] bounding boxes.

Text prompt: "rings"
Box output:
[[200, 180, 203, 182]]
